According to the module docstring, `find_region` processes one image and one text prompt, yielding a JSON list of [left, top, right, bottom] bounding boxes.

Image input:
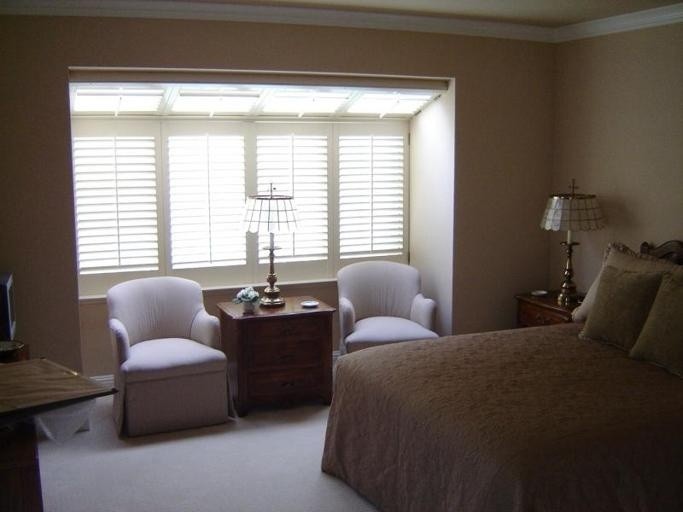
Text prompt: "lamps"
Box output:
[[241, 181, 300, 307], [536, 177, 607, 303]]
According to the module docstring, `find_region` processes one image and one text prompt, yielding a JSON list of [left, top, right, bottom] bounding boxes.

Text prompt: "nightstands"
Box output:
[[515, 292, 584, 329], [214, 296, 336, 420]]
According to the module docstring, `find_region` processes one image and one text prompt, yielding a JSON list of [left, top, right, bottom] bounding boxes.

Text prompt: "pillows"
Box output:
[[571, 240, 682, 378]]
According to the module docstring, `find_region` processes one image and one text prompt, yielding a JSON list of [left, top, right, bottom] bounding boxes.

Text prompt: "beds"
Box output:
[[333, 238, 683, 511]]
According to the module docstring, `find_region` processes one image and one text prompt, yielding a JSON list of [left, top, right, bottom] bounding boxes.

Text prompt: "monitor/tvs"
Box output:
[[0, 271, 18, 342]]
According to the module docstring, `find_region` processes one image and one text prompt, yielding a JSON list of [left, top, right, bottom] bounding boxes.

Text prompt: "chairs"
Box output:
[[335, 261, 440, 355], [104, 275, 229, 440]]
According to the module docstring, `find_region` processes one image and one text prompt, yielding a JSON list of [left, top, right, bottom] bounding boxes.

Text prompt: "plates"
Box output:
[[301, 301, 319, 307], [531, 290, 548, 295]]
